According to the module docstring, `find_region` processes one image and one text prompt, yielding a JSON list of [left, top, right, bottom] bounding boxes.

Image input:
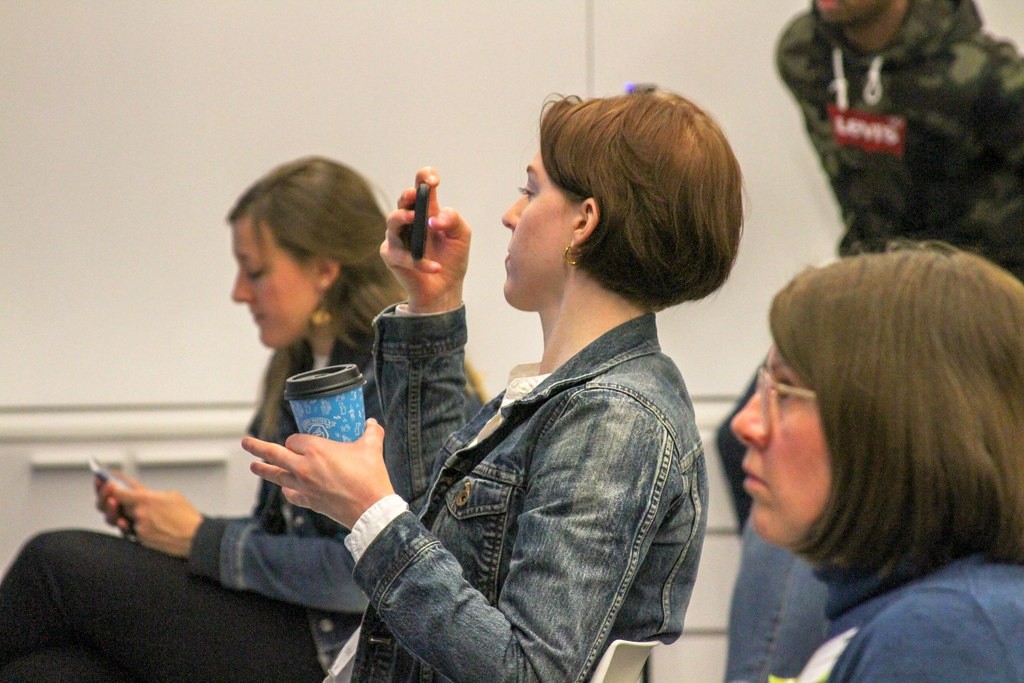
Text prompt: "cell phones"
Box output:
[[410, 183, 430, 259], [90, 459, 130, 491]]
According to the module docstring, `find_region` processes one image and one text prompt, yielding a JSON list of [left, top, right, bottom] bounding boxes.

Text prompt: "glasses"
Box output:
[[756, 368, 817, 415]]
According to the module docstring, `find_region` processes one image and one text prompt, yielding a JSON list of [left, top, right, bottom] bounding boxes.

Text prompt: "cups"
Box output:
[[283, 364, 366, 442]]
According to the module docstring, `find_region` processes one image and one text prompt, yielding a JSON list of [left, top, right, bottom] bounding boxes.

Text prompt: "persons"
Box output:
[[717, 0, 1024, 681], [1, 155, 486, 683], [245, 83, 741, 681]]
